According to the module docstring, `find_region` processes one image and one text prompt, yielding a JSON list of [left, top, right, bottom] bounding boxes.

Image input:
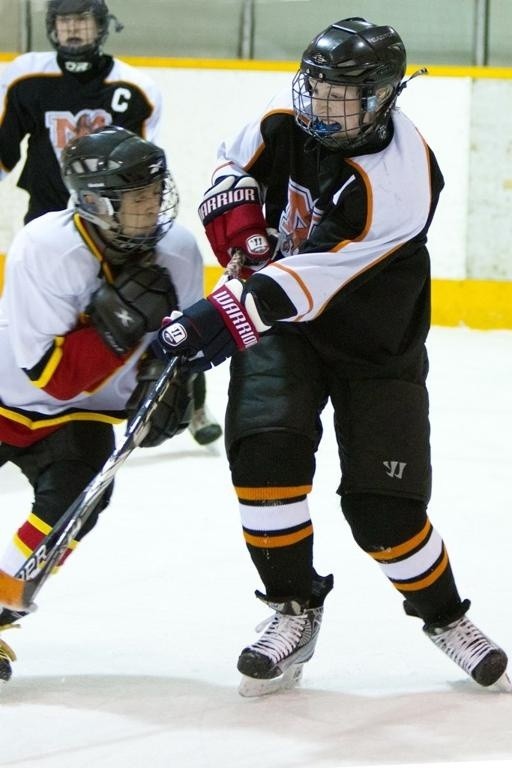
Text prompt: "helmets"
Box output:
[[45, 0, 110, 62], [60, 124, 181, 254], [291, 15, 408, 154]]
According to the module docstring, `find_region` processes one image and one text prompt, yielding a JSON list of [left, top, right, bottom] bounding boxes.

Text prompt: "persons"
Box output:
[[158, 13, 508, 690], [0, 0, 165, 225], [1, 124, 208, 685]]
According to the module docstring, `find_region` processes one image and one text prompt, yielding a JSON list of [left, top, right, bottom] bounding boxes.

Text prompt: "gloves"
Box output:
[[124, 354, 195, 448], [197, 174, 284, 285], [151, 277, 278, 377], [83, 247, 180, 355]]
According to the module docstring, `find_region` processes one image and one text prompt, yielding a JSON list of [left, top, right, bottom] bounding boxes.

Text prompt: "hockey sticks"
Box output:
[[1, 251, 245, 612]]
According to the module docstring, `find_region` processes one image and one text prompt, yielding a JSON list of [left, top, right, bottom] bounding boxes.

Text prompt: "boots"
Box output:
[[0, 601, 39, 681], [234, 565, 335, 699], [403, 598, 512, 694], [182, 371, 223, 457]]
